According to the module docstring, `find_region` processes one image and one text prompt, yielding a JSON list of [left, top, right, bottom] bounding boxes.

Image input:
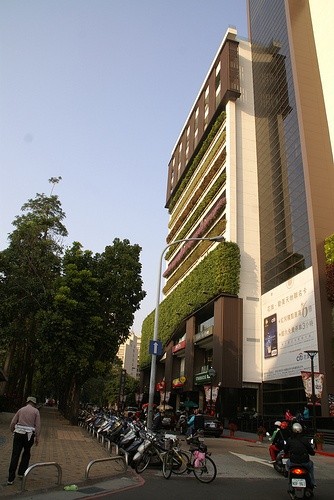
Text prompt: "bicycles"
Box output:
[[79, 402, 218, 483]]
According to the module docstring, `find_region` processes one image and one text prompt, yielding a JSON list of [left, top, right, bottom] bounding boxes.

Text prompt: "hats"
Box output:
[[26, 397, 37, 403]]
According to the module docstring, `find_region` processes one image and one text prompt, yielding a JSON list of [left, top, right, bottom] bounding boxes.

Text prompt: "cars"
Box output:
[[201, 415, 227, 437]]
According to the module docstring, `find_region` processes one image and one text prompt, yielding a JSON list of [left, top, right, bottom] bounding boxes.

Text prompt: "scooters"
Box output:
[[283, 438, 318, 500], [266, 432, 291, 477]]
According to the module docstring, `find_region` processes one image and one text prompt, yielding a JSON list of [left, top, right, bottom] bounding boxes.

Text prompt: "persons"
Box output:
[[285, 409, 294, 421], [186, 412, 195, 440], [303, 407, 309, 426], [268, 421, 291, 464], [8, 397, 41, 485], [286, 423, 318, 487]]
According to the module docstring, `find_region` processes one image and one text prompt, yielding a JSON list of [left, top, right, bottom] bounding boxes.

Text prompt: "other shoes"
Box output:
[[17, 475, 23, 478], [268, 460, 276, 464], [8, 478, 16, 484]]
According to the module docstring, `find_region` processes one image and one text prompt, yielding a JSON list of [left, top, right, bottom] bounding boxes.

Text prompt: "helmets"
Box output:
[[274, 421, 281, 426], [281, 421, 289, 427], [293, 423, 303, 433]]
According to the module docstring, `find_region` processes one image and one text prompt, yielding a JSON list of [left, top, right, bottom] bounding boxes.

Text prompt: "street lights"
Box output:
[[303, 350, 319, 435], [145, 235, 226, 431]]
